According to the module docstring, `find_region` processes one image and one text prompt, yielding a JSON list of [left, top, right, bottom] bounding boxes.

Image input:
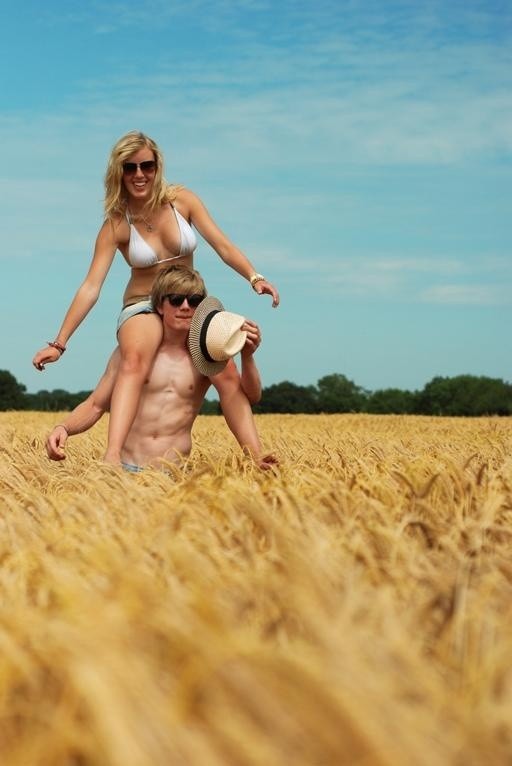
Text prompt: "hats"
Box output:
[[188, 297, 248, 377]]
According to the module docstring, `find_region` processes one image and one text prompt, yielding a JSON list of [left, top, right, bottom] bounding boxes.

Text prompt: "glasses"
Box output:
[[120, 160, 157, 175], [160, 294, 205, 307]]
